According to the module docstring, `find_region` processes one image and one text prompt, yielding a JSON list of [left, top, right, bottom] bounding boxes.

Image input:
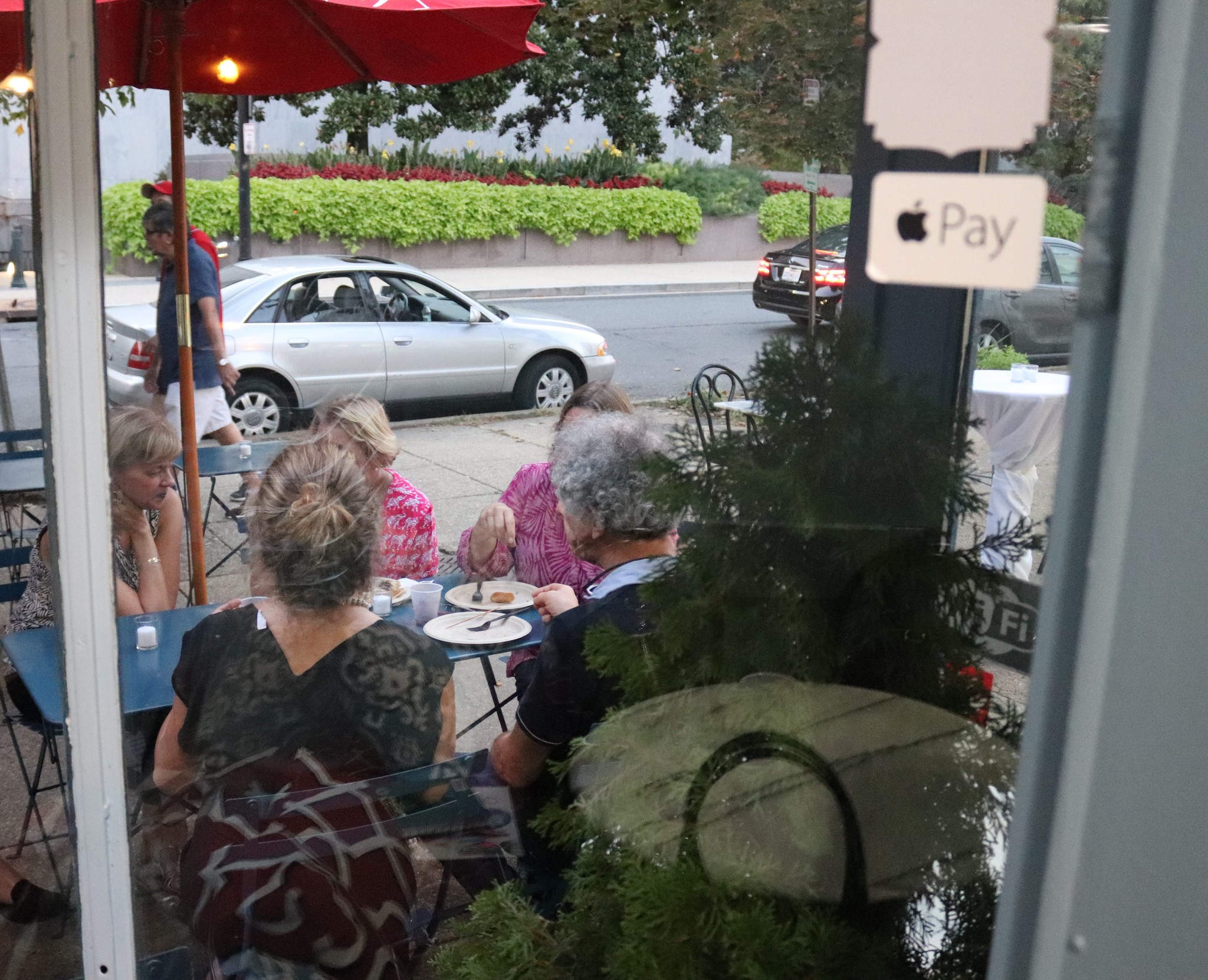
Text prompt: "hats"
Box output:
[[141, 180, 173, 199]]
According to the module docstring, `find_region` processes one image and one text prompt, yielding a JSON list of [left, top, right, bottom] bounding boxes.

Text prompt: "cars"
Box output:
[[752, 223, 853, 327], [106, 254, 616, 438], [976, 236, 1083, 364]]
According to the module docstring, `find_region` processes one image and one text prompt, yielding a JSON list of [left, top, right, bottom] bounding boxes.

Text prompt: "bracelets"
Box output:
[[137, 554, 161, 568]]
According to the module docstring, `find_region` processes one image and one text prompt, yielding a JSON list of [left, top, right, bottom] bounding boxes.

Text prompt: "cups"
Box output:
[[1011, 363, 1024, 382], [238, 440, 252, 460], [1024, 364, 1039, 380], [133, 615, 159, 651], [370, 586, 392, 617], [238, 597, 270, 630], [410, 583, 443, 626]]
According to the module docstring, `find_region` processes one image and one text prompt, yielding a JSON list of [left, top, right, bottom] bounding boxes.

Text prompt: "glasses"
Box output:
[[144, 228, 162, 238]]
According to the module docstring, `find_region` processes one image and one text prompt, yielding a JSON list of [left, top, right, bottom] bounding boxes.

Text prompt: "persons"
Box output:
[[307, 395, 440, 581], [0, 400, 186, 788], [141, 179, 260, 502], [456, 380, 632, 703], [489, 412, 696, 920], [133, 441, 459, 980]]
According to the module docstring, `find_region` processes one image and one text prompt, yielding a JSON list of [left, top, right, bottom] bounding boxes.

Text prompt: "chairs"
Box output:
[[322, 285, 366, 322], [0, 428, 490, 980], [691, 364, 761, 471], [293, 287, 328, 320]]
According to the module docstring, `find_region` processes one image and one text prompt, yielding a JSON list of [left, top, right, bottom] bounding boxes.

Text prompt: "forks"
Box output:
[[466, 607, 530, 631], [472, 578, 483, 603]]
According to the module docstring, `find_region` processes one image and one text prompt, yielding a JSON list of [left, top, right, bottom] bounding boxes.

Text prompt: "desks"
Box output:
[[0, 456, 45, 620], [969, 369, 1070, 580], [172, 443, 292, 607], [0, 573, 552, 903]]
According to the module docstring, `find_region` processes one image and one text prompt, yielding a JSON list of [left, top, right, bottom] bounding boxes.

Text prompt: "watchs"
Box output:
[[215, 358, 230, 366]]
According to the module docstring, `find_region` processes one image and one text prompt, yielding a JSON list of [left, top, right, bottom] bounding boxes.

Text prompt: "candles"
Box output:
[[239, 440, 251, 459], [134, 615, 159, 651], [369, 587, 392, 618], [1023, 365, 1038, 382], [1010, 363, 1023, 383]]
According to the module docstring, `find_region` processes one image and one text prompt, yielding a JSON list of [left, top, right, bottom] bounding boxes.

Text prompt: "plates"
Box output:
[[422, 611, 532, 644], [368, 576, 412, 604], [446, 580, 540, 611]]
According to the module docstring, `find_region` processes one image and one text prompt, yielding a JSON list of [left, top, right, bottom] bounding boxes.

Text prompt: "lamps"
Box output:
[[0, 55, 33, 94]]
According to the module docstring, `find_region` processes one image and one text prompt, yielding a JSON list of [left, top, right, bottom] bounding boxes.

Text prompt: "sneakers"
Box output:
[[241, 486, 241, 486], [415, 779, 512, 830], [225, 492, 257, 518]]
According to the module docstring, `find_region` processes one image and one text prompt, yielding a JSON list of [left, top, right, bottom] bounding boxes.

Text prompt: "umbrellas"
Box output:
[[0, 0, 553, 610]]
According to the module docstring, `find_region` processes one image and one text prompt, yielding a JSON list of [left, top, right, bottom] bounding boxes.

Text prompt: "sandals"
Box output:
[[155, 833, 185, 893], [134, 854, 181, 908]]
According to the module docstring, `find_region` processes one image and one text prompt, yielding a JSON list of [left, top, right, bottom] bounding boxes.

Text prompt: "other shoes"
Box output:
[[229, 482, 248, 502], [0, 879, 69, 924]]
